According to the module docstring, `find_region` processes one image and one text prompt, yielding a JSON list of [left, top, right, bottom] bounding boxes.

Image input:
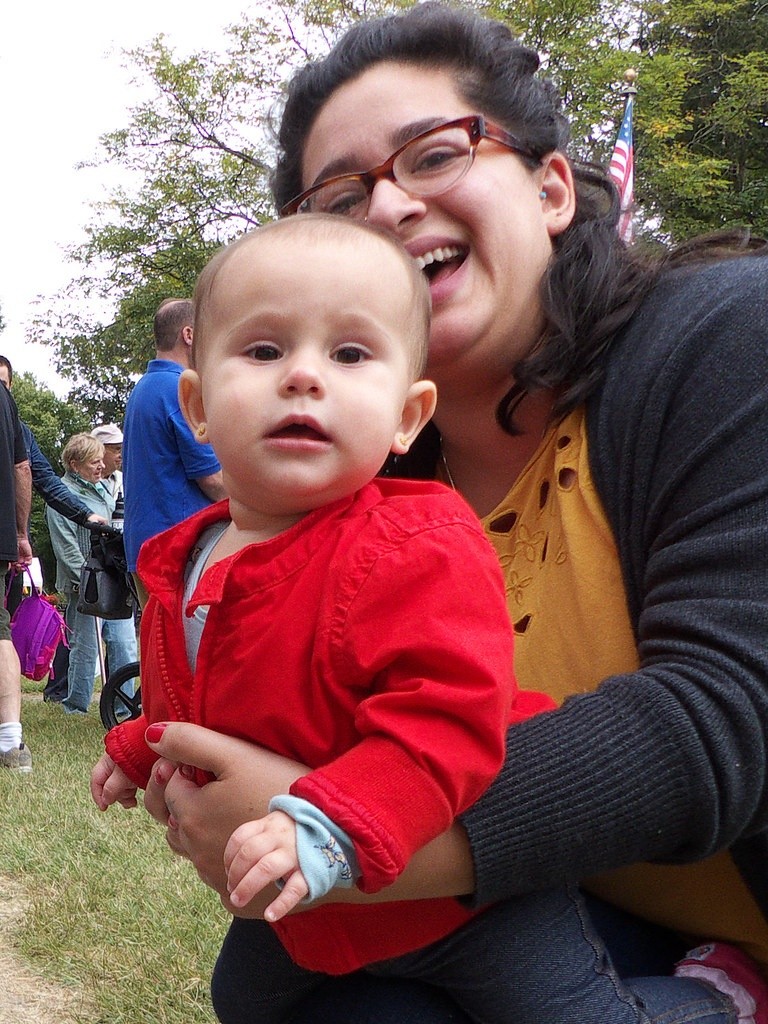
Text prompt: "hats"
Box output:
[[90, 425, 125, 444]]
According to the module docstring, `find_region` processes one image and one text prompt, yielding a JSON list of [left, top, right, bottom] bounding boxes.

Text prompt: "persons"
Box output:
[[93, 210, 768, 1024], [264, 9, 768, 1024], [0, 302, 227, 776]]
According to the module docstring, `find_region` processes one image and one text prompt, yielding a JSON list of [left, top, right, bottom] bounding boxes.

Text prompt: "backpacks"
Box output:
[[3, 563, 71, 682]]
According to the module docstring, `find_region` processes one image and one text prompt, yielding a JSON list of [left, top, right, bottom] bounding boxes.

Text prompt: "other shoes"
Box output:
[[0, 741, 32, 773], [680, 943, 768, 1024]]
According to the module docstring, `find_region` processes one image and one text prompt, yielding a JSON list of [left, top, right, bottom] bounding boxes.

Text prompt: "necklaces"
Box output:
[[441, 390, 559, 498]]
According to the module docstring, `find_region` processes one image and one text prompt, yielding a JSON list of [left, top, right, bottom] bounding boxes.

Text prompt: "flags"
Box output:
[[610, 93, 637, 240]]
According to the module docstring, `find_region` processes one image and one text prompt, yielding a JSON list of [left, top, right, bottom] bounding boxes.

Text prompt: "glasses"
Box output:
[[280, 113, 542, 220]]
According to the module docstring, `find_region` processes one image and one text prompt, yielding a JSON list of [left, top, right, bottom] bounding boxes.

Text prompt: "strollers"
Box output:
[[83, 522, 142, 732]]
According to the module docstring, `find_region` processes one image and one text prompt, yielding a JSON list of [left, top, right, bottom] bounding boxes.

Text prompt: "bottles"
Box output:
[[111, 498, 125, 533]]
